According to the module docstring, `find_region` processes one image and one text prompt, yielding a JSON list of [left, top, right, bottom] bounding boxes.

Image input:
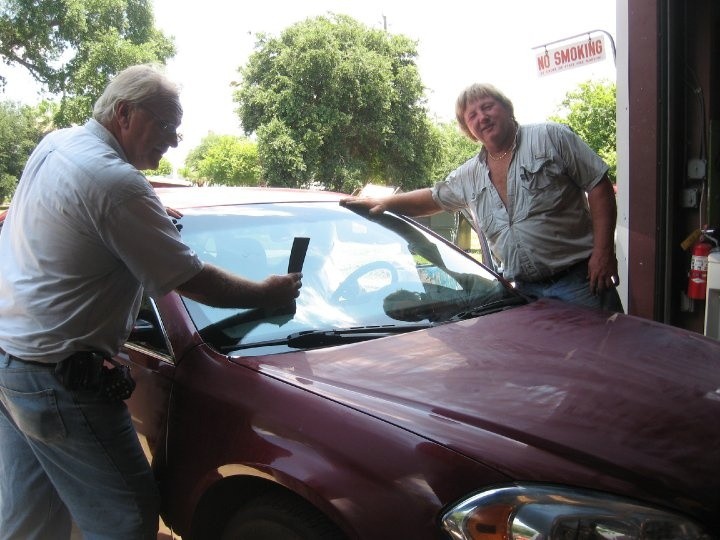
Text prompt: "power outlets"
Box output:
[[687, 159, 707, 179], [678, 188, 698, 210]]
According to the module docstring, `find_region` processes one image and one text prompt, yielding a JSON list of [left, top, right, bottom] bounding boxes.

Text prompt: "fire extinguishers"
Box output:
[[687, 230, 718, 299]]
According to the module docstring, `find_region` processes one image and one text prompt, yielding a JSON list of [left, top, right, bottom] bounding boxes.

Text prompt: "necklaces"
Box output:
[[487, 129, 517, 160]]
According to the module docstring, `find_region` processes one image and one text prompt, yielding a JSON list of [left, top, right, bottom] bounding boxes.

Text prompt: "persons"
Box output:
[[0, 66, 303, 540], [340, 83, 625, 314]]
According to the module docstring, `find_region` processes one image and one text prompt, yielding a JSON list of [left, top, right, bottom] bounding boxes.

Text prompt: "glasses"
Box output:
[[144, 105, 183, 143]]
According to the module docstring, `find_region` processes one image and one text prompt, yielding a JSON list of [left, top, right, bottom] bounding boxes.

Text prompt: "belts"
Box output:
[[540, 257, 588, 288], [0, 347, 57, 367]]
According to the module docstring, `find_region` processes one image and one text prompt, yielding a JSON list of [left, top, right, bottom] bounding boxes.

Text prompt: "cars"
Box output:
[[0, 186, 720, 540]]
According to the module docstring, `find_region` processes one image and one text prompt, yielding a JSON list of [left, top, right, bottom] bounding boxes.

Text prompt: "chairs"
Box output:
[[278, 239, 336, 302], [220, 237, 270, 281]]
